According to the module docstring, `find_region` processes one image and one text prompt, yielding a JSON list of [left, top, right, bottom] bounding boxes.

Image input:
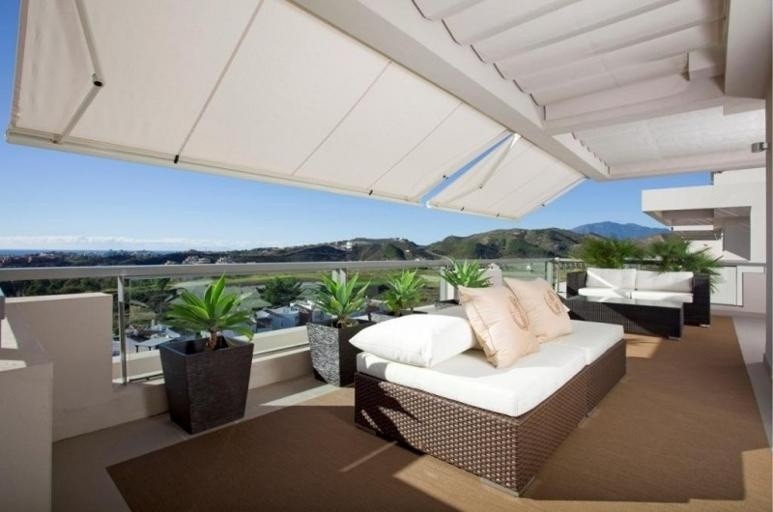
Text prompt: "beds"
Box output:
[[352, 321, 626, 498]]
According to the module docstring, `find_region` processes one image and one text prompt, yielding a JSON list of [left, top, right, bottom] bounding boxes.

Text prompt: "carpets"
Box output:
[[105, 314, 772, 511]]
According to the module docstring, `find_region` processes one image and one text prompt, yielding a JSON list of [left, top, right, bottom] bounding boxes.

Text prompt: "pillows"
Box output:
[[347, 277, 574, 368]]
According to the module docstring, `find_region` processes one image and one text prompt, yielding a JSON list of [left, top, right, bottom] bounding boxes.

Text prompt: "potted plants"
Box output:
[[306, 266, 376, 385], [159, 272, 262, 433], [435, 259, 498, 303], [380, 259, 432, 316]]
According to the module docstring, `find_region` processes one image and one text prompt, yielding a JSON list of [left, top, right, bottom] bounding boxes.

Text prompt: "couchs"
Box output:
[[565, 267, 710, 327]]
